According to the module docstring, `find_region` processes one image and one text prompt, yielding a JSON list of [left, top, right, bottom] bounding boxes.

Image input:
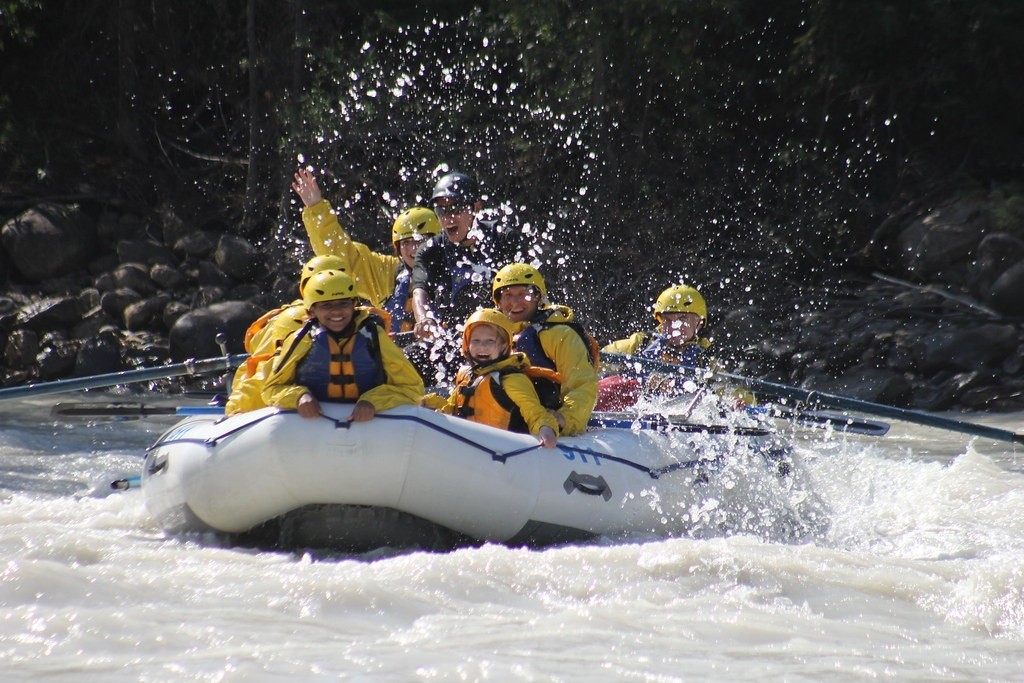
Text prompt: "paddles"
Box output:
[[769, 406, 890, 436], [599, 347, 1024, 448], [30, 400, 225, 420], [1, 329, 415, 398]]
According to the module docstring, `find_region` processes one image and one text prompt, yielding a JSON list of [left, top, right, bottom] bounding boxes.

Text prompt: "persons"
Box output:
[[419, 308, 559, 448], [597, 284, 759, 414], [483, 262, 600, 436], [291, 168, 444, 353], [211, 254, 355, 427], [260, 269, 425, 422], [403, 172, 530, 394]]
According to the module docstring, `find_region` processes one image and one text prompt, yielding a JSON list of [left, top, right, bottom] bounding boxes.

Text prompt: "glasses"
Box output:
[[435, 201, 469, 216]]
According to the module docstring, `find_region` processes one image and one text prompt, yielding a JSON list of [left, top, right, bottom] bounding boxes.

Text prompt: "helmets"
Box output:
[[299, 255, 351, 298], [655, 285, 707, 328], [303, 269, 358, 310], [428, 173, 479, 204], [493, 263, 546, 308], [392, 207, 442, 249], [462, 308, 512, 357]]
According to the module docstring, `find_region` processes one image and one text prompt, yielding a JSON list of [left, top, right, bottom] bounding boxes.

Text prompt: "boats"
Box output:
[[140, 388, 835, 552]]
